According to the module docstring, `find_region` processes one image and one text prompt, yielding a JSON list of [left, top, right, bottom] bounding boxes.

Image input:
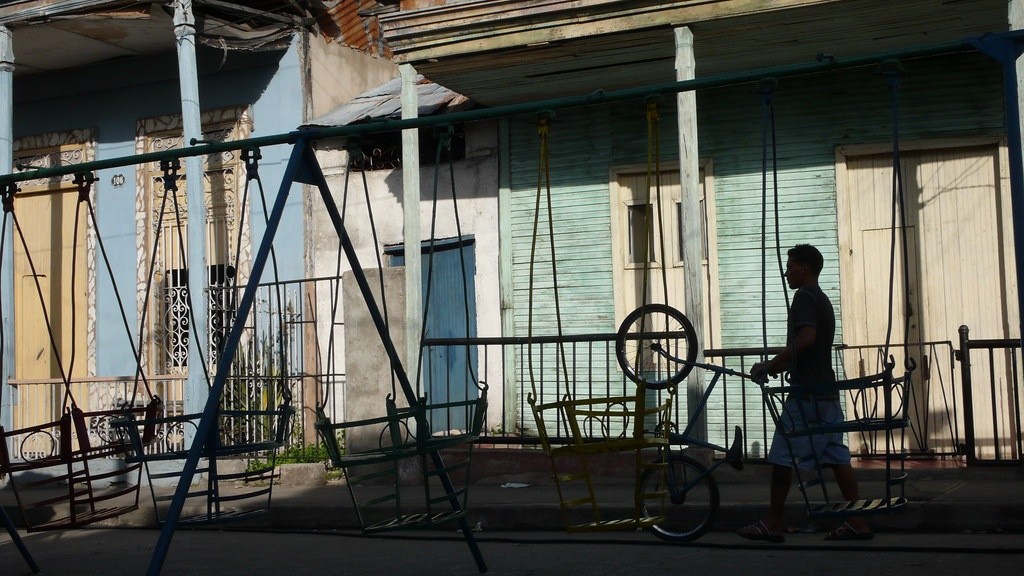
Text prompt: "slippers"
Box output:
[[824, 521, 874, 540], [737, 521, 785, 541]]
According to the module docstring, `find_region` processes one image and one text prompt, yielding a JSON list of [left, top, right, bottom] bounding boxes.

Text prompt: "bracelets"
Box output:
[[763, 359, 779, 379]]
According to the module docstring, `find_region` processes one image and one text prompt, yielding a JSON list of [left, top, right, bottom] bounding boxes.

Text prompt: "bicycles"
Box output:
[[615, 302, 774, 545]]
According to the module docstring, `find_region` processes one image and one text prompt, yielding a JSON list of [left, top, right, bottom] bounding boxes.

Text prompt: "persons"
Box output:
[[738, 243, 875, 545]]
